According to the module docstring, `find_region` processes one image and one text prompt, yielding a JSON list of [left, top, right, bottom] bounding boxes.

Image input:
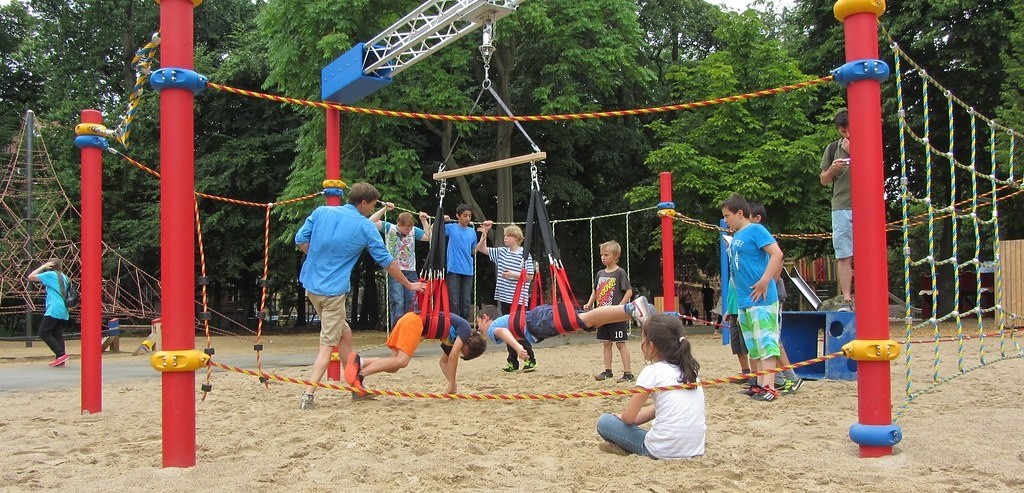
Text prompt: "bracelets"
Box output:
[[42, 265, 46, 269]]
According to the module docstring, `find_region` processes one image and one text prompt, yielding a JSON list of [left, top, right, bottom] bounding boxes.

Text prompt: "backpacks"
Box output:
[[64, 279, 80, 308]]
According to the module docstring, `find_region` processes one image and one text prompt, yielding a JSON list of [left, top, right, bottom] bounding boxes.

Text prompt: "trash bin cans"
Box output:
[[992, 240, 1024, 328], [654, 297, 679, 313]]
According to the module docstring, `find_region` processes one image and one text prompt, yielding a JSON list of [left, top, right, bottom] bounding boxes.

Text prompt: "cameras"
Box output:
[[841, 158, 850, 165]]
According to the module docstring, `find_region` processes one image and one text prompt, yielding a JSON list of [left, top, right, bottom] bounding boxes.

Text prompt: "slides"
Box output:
[[781, 263, 824, 311], [852, 269, 923, 313]]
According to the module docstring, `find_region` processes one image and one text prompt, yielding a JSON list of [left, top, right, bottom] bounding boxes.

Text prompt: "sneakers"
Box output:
[[630, 295, 659, 327], [501, 360, 519, 372], [780, 375, 805, 397], [522, 357, 538, 373], [772, 379, 788, 390]]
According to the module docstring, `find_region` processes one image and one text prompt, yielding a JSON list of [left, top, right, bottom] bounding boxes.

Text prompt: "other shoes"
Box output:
[[740, 373, 758, 389], [747, 384, 779, 402], [594, 371, 614, 380], [343, 351, 361, 385], [837, 299, 853, 312], [738, 384, 763, 397], [350, 389, 378, 401], [617, 373, 636, 383], [298, 390, 316, 410], [48, 355, 71, 367], [352, 374, 364, 390]]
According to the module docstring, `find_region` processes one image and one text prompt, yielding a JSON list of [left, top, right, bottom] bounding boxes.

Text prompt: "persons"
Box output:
[[479, 221, 537, 373], [369, 202, 431, 327], [344, 311, 487, 394], [710, 297, 722, 334], [722, 196, 805, 402], [430, 205, 486, 320], [477, 296, 657, 360], [596, 314, 706, 460], [584, 241, 635, 382], [680, 287, 693, 326], [702, 282, 716, 325], [295, 182, 427, 409], [28, 259, 69, 366], [820, 110, 854, 312]]
